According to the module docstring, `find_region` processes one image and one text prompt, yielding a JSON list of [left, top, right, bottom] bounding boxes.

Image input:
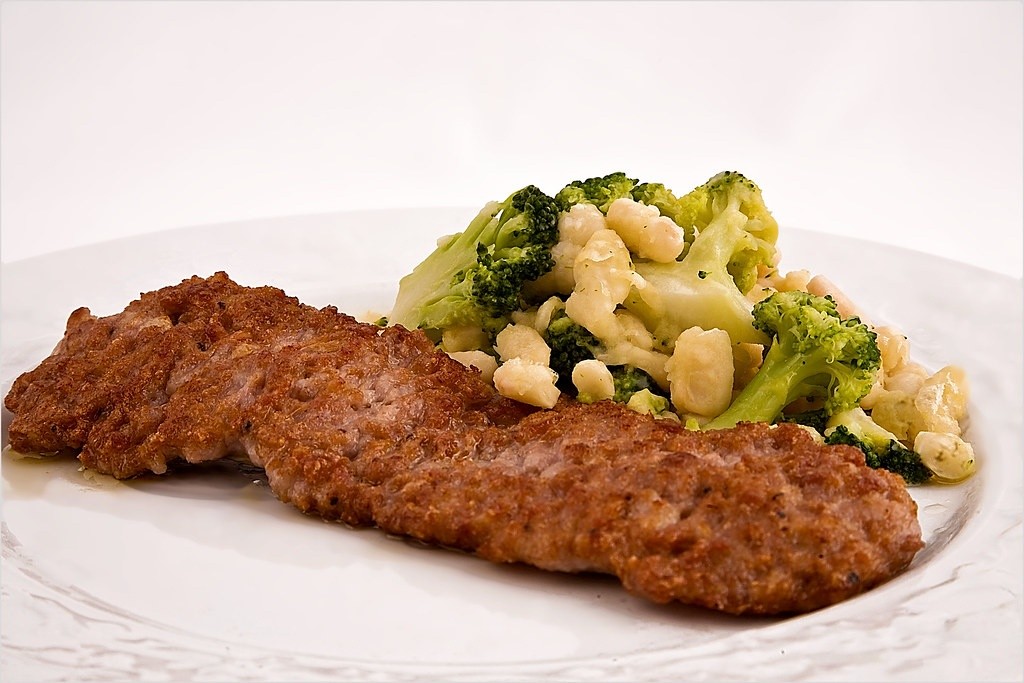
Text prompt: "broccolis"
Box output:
[[371, 171, 934, 489]]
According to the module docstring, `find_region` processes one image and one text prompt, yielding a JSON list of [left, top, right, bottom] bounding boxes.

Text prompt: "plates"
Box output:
[[0, 208, 1024, 682]]
